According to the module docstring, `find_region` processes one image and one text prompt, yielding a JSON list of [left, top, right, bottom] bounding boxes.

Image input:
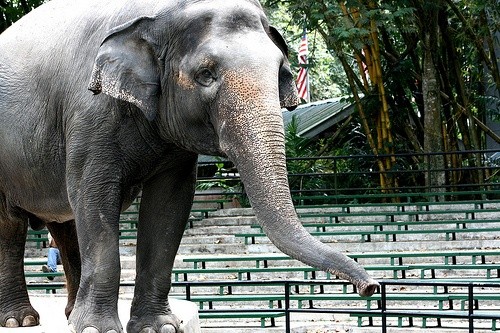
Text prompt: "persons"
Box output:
[[42, 232, 64, 280]]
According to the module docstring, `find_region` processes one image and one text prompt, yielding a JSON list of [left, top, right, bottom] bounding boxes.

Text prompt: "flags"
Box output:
[[294, 33, 307, 105]]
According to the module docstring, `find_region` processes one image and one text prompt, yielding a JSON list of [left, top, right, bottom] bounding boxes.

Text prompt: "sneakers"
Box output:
[[42, 265, 55, 280]]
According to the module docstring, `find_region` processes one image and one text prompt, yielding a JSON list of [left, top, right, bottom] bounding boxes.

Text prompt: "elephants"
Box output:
[[0, 0, 381, 333]]
